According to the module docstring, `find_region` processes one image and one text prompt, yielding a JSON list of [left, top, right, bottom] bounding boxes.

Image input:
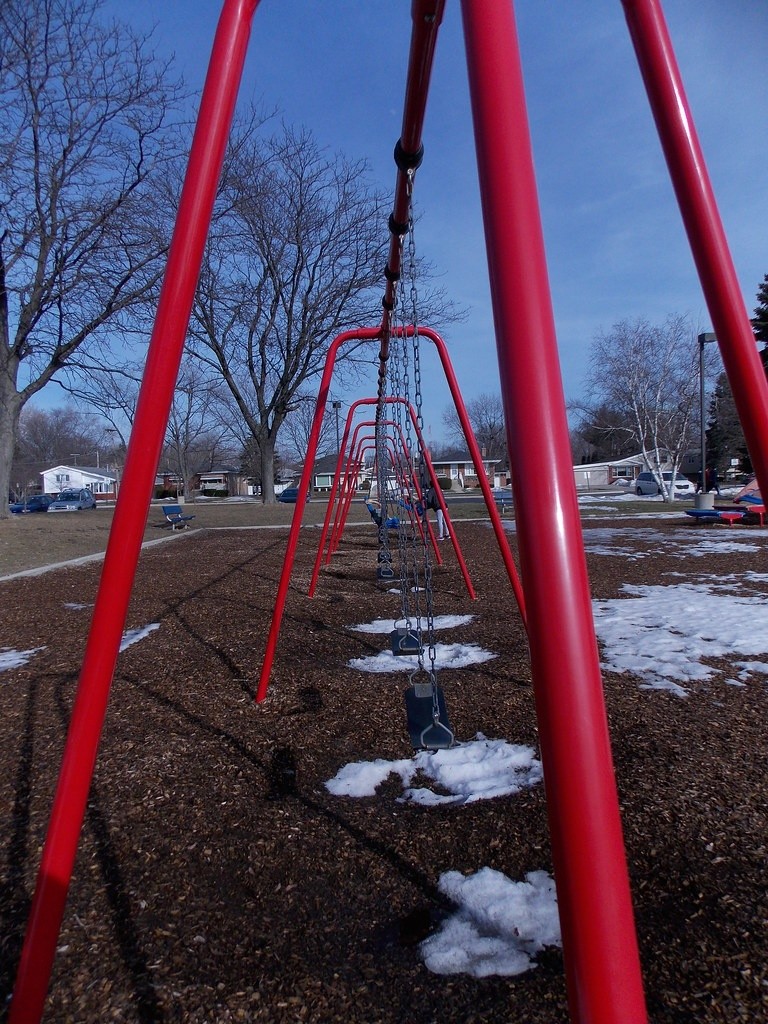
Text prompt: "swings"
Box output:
[[397, 162, 458, 755], [364, 234, 426, 659]]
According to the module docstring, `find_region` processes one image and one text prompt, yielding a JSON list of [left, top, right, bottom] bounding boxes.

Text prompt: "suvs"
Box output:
[[48, 487, 96, 512]]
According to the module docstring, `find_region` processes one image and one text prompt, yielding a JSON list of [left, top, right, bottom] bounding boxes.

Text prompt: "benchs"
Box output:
[[163, 506, 196, 531], [685, 510, 743, 527], [492, 491, 513, 514]]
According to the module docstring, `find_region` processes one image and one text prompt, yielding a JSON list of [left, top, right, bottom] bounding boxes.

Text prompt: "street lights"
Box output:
[[698, 332, 717, 495], [333, 402, 341, 499]]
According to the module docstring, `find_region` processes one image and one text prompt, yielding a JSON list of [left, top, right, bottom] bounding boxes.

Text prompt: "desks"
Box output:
[[712, 505, 766, 527]]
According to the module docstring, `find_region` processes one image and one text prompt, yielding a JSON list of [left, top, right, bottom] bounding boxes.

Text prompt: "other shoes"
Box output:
[[444, 534, 451, 539], [435, 536, 444, 541]]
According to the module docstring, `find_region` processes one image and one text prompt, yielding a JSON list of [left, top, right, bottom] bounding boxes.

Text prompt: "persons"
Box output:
[[695, 465, 723, 495], [427, 481, 451, 540]]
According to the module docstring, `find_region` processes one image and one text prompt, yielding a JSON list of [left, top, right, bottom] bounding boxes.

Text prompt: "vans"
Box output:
[[636, 470, 695, 496], [276, 487, 311, 503]]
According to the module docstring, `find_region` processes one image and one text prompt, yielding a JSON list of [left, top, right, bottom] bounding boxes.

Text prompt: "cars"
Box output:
[[9, 495, 18, 503], [740, 475, 755, 485], [360, 481, 372, 490], [9, 495, 54, 513]]
